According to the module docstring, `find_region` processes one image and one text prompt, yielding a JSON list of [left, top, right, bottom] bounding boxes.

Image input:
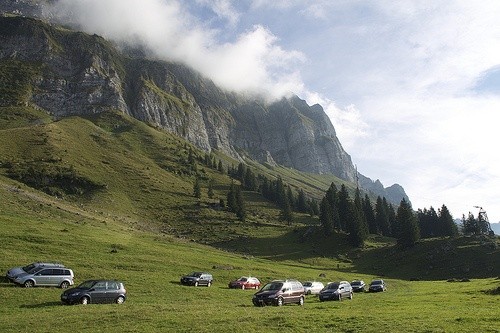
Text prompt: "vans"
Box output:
[[6, 263, 74, 289]]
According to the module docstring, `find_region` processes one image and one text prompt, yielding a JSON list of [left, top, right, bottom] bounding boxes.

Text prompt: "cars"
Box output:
[[304, 280, 325, 294], [350, 280, 366, 292], [369, 280, 386, 292]]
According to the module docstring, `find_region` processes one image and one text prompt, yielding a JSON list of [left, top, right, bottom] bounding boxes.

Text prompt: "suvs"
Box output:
[[181, 271, 213, 285], [229, 276, 261, 290], [251, 280, 307, 307], [60, 279, 128, 307], [319, 280, 355, 299]]
